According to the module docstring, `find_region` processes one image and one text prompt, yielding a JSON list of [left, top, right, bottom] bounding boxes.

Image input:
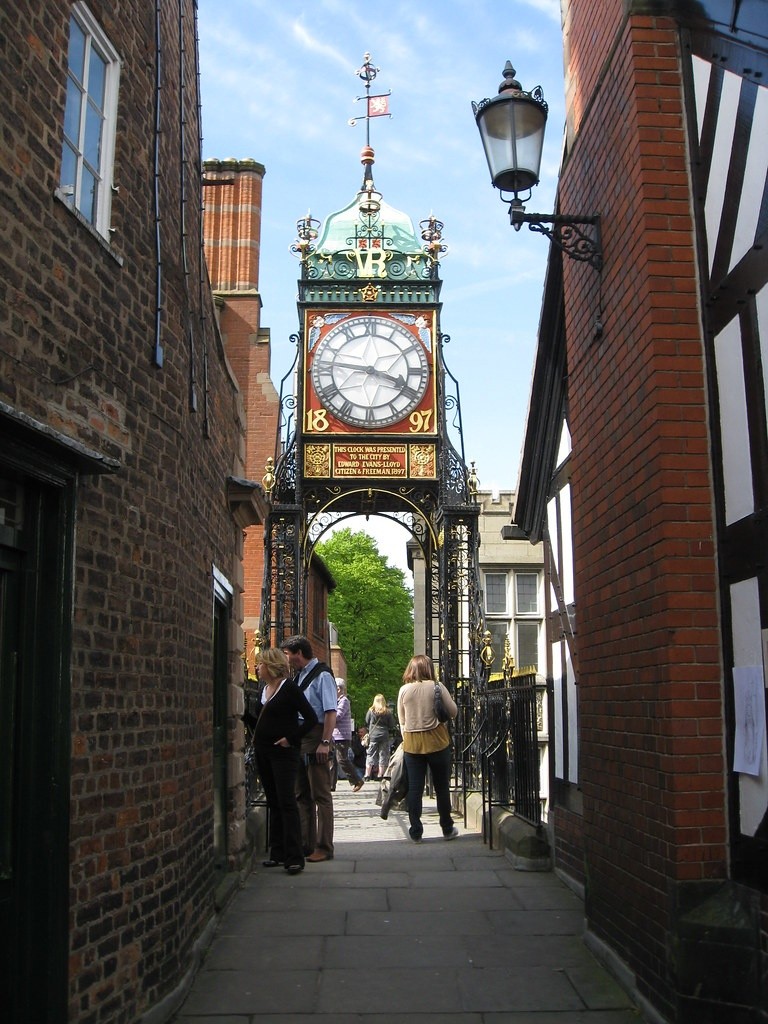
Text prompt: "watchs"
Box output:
[[320, 739, 331, 745]]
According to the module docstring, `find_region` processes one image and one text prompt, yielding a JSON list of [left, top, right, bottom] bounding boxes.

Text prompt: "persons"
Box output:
[[329, 677, 364, 791], [362, 693, 395, 781], [334, 727, 369, 780], [242, 647, 319, 873], [281, 636, 338, 863], [396, 655, 459, 844]]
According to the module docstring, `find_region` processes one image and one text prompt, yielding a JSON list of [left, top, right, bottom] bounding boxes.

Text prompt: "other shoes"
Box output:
[[362, 776, 370, 781], [375, 776, 382, 781], [444, 827, 458, 839], [412, 837, 423, 844]]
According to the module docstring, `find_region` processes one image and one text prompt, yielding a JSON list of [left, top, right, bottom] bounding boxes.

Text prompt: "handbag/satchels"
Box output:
[[361, 734, 370, 747], [245, 747, 255, 771], [434, 680, 448, 724]]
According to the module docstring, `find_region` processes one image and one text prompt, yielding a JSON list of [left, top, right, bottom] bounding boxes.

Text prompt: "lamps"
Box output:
[[472, 59, 601, 271]]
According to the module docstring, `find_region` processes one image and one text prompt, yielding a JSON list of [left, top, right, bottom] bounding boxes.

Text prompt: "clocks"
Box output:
[[310, 315, 431, 430]]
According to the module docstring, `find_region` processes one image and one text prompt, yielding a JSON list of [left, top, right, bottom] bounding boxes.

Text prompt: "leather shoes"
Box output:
[[352, 779, 364, 792], [262, 860, 278, 867], [288, 864, 301, 873], [306, 851, 330, 862], [331, 788, 335, 791]]
